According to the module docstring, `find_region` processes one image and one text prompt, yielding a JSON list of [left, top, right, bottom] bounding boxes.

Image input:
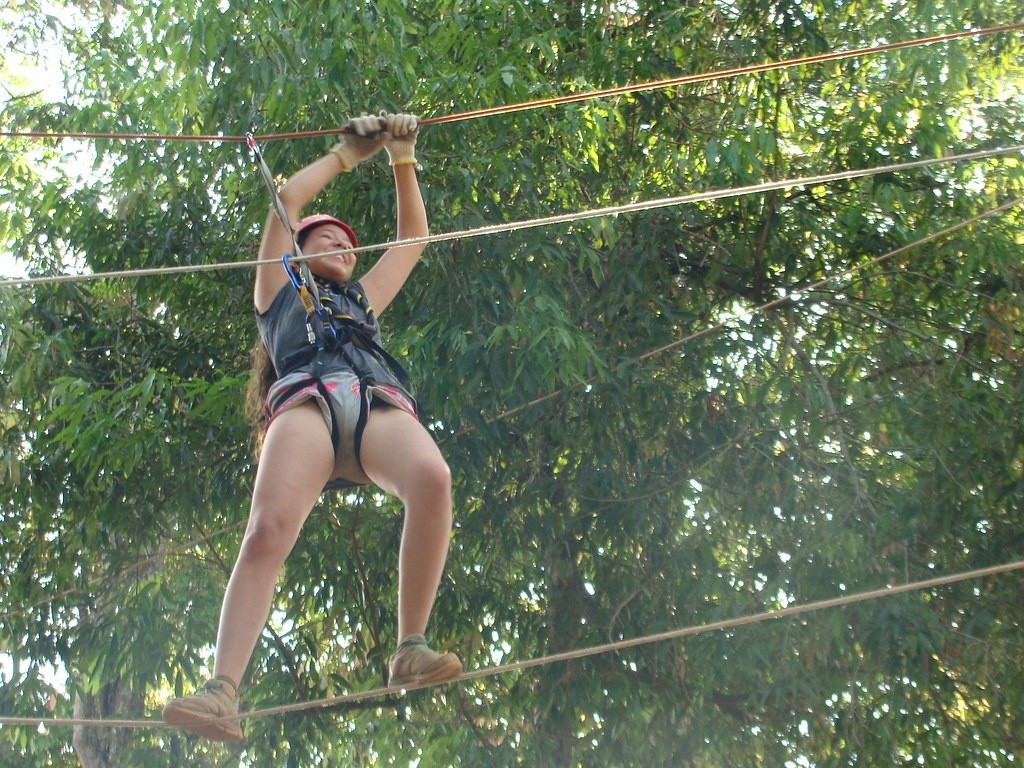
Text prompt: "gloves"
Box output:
[[379, 109, 421, 166], [328, 110, 390, 172]]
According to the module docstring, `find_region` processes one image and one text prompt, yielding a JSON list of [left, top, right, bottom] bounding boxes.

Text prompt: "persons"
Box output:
[[161, 114, 463, 744]]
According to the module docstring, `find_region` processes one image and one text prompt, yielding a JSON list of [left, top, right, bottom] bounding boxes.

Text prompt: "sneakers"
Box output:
[[386, 631, 461, 688], [163, 678, 243, 744]]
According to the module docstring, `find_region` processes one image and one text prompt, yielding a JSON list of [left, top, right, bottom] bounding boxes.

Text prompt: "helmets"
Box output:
[[291, 214, 359, 255]]
